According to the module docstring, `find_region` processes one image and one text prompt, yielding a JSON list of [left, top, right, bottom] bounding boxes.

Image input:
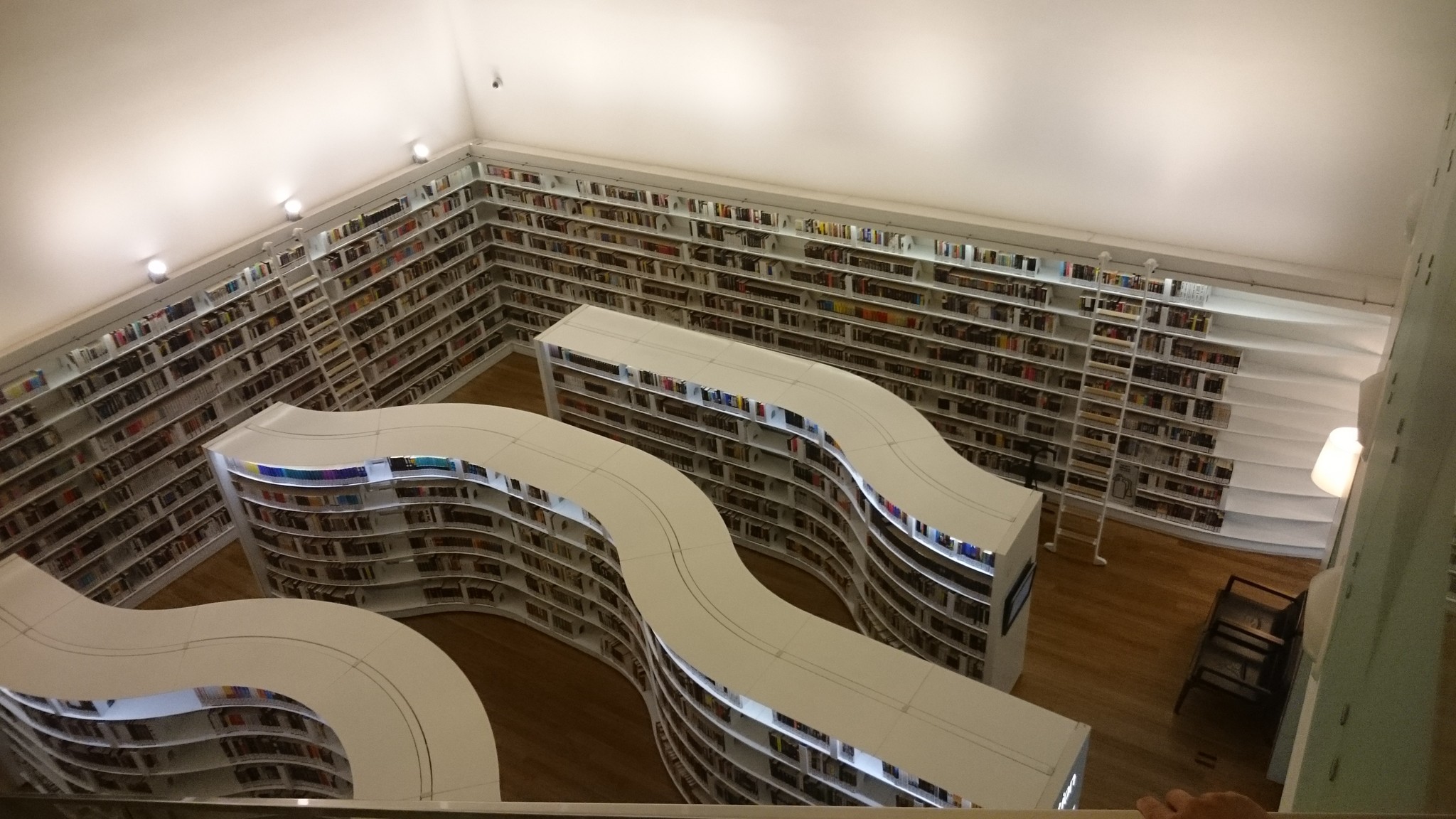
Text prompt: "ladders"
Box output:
[[263, 228, 377, 412], [1045, 252, 1158, 567]]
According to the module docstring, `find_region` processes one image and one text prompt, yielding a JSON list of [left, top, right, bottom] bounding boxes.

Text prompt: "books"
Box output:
[[0, 152, 1242, 818]]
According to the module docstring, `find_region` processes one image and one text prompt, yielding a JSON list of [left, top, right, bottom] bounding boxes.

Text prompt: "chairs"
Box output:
[[1199, 574, 1308, 643], [1173, 619, 1303, 740]]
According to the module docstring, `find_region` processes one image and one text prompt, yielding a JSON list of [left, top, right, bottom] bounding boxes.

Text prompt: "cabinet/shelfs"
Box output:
[[0, 141, 1403, 819]]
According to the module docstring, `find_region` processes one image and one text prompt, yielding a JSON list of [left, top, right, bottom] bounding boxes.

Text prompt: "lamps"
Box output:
[[146, 260, 194, 287], [413, 143, 430, 165], [1311, 427, 1363, 498], [283, 200, 313, 221]]
